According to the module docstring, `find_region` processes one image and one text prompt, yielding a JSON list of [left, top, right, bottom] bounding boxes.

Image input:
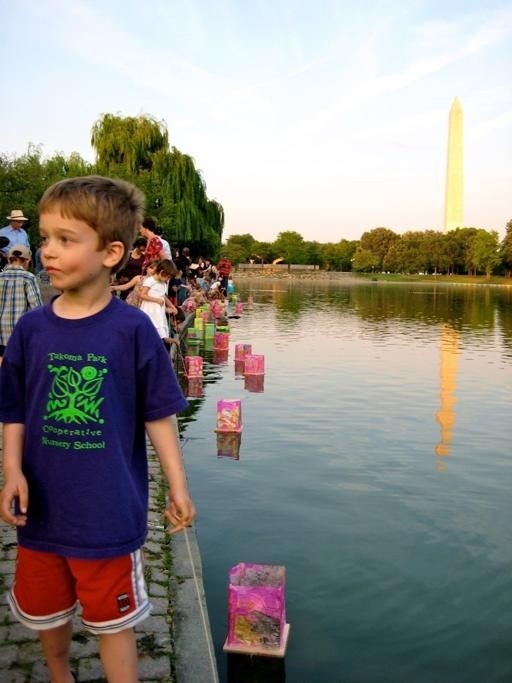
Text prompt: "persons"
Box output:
[[0, 174, 201, 683], [1, 205, 236, 369]]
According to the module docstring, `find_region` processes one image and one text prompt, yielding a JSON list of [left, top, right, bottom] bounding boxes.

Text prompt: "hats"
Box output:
[[6, 210, 29, 221], [7, 243, 32, 260]]
